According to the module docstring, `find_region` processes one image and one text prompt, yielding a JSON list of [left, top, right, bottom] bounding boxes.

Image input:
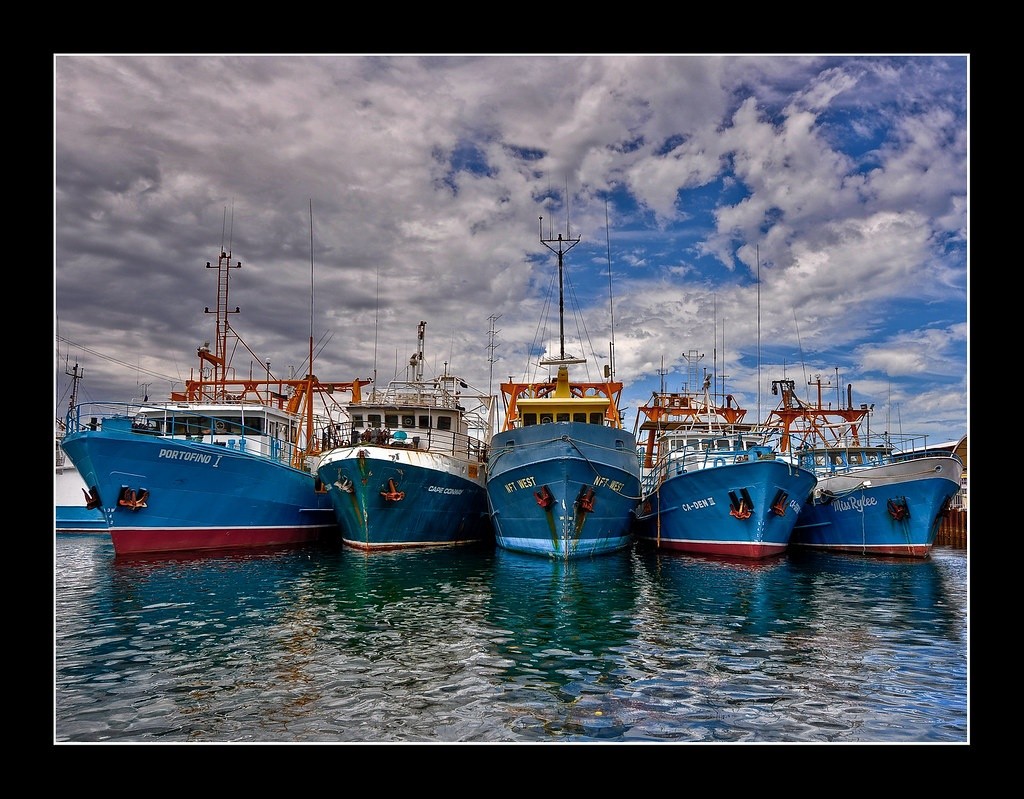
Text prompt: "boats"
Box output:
[[53, 170, 966, 559]]
